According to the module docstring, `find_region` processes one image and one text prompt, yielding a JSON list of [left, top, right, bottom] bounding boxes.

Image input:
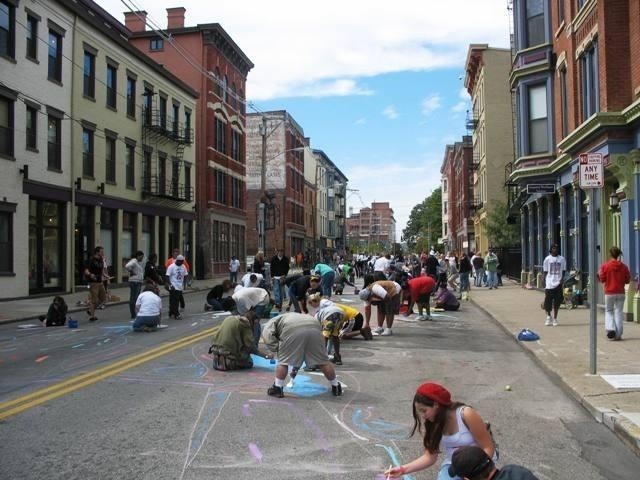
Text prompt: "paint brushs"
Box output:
[[286, 371, 298, 388], [270, 357, 275, 364], [386, 464, 393, 480]]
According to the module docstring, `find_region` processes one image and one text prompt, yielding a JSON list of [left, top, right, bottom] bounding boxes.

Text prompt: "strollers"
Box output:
[[540, 270, 581, 310]]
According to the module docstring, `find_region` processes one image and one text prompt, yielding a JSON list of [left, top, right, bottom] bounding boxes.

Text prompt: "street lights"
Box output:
[[263, 147, 359, 245]]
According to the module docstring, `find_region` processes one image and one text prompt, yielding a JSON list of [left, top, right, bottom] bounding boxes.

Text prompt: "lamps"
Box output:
[[609, 192, 619, 211]]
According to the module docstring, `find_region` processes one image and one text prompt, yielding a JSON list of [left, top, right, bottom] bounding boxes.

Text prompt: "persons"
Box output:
[[84, 248, 111, 322], [542, 244, 567, 327], [44, 296, 68, 328], [449, 446, 539, 480], [598, 247, 631, 341], [384, 383, 498, 480], [207, 249, 499, 397], [125, 249, 191, 332]]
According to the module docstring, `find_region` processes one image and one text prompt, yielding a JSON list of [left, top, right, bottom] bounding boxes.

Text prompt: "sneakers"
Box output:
[[415, 315, 432, 321], [329, 353, 342, 365], [361, 326, 392, 340], [268, 381, 284, 398], [545, 315, 559, 326], [332, 382, 342, 395]]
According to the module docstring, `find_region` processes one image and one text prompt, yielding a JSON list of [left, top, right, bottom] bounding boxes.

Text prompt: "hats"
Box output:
[[416, 383, 452, 405], [358, 287, 370, 300], [448, 446, 490, 477]]
